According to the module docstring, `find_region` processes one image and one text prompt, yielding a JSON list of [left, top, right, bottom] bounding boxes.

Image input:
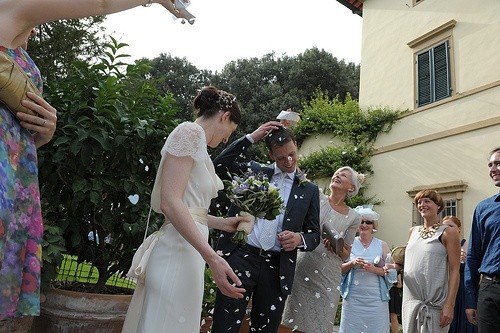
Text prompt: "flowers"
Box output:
[[298, 175, 312, 187], [228, 173, 284, 241]]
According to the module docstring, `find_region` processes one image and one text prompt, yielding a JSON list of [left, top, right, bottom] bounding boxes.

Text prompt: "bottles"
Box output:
[[460, 246, 465, 264], [385, 253, 397, 283]]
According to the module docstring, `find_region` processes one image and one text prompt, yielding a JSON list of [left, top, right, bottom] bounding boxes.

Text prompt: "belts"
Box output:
[[239, 243, 279, 259], [484, 274, 500, 283]]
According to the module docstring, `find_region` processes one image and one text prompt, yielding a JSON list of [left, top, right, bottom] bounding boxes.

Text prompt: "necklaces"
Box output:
[[419, 221, 441, 239], [359, 238, 369, 246]]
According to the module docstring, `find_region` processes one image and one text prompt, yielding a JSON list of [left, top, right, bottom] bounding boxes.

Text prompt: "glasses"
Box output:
[[361, 220, 373, 225]]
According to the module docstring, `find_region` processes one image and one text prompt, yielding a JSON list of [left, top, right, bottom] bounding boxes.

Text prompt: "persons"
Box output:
[[463, 148, 500, 333], [339, 205, 397, 333], [212, 121, 320, 333], [383, 189, 461, 333], [0, 0, 184, 333], [121, 86, 246, 333], [388, 262, 403, 333], [278, 166, 363, 333], [440, 215, 478, 333]]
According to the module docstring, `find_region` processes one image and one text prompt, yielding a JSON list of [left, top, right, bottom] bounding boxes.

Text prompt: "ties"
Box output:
[[259, 173, 286, 251]]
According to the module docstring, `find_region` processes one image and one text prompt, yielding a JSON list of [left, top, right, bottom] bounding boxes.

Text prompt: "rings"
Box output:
[[41, 120, 46, 126], [286, 244, 288, 248]]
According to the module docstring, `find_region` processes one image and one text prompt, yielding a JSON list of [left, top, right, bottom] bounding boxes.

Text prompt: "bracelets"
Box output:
[[142, 0, 152, 7]]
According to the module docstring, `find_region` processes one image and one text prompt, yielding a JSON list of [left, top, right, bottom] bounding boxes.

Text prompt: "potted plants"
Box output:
[[32, 36, 187, 333]]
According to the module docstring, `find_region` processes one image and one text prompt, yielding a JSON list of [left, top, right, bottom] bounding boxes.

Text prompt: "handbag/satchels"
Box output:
[[0, 51, 42, 136], [322, 223, 344, 254], [391, 246, 405, 265]]
